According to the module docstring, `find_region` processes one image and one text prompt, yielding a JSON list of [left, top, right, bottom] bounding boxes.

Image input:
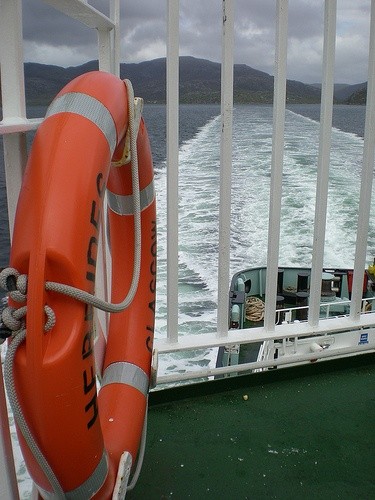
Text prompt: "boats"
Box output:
[[213, 266, 375, 379]]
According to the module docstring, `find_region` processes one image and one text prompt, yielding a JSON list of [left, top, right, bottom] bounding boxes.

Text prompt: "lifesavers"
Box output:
[[9, 72, 157, 500]]
